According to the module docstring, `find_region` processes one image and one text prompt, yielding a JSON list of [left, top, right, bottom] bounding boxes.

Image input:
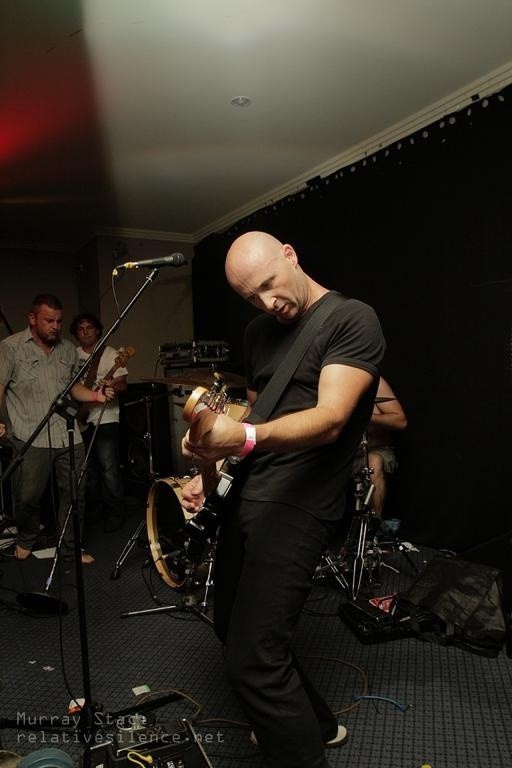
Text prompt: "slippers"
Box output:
[[250, 725, 349, 748]]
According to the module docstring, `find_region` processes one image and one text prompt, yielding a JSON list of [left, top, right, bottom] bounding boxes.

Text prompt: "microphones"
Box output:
[[160, 548, 182, 562], [117, 253, 184, 267]]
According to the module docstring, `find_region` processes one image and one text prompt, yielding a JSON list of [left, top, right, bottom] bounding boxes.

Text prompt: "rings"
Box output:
[[191, 452, 196, 461]]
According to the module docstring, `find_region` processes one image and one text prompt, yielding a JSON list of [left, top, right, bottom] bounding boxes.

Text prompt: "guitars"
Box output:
[[76, 346, 135, 433], [183, 379, 233, 567]]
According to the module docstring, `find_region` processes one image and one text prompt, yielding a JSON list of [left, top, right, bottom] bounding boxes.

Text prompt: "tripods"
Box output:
[[120, 561, 214, 629], [0, 267, 183, 768], [324, 431, 425, 601]]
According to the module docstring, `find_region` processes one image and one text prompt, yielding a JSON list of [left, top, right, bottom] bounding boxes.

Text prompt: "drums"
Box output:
[[146, 476, 222, 592], [225, 397, 247, 421]]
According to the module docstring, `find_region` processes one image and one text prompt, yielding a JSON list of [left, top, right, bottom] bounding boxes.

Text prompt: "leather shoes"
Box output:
[[103, 515, 125, 532]]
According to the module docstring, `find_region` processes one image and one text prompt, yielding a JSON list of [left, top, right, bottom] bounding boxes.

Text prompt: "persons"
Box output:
[[67, 314, 137, 533], [353, 376, 408, 517], [0, 293, 115, 587], [181, 232, 386, 767]]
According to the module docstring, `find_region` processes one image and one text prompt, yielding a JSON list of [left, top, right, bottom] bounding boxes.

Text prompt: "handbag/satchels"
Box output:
[[400, 554, 507, 657]]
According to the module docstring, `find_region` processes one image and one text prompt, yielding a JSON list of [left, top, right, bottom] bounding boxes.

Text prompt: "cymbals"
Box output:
[[140, 369, 260, 386]]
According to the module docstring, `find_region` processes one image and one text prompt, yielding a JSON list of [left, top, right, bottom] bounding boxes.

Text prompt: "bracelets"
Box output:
[[93, 390, 98, 401], [239, 422, 258, 458]]
